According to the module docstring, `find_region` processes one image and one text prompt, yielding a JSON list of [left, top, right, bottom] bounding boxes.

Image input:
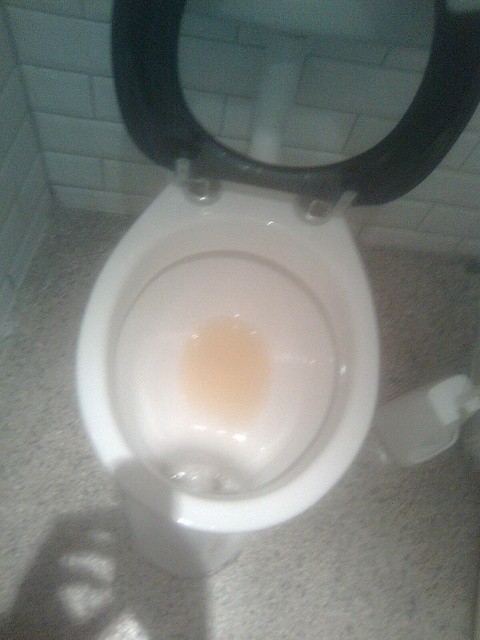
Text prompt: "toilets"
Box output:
[[73, 0, 480, 585]]
